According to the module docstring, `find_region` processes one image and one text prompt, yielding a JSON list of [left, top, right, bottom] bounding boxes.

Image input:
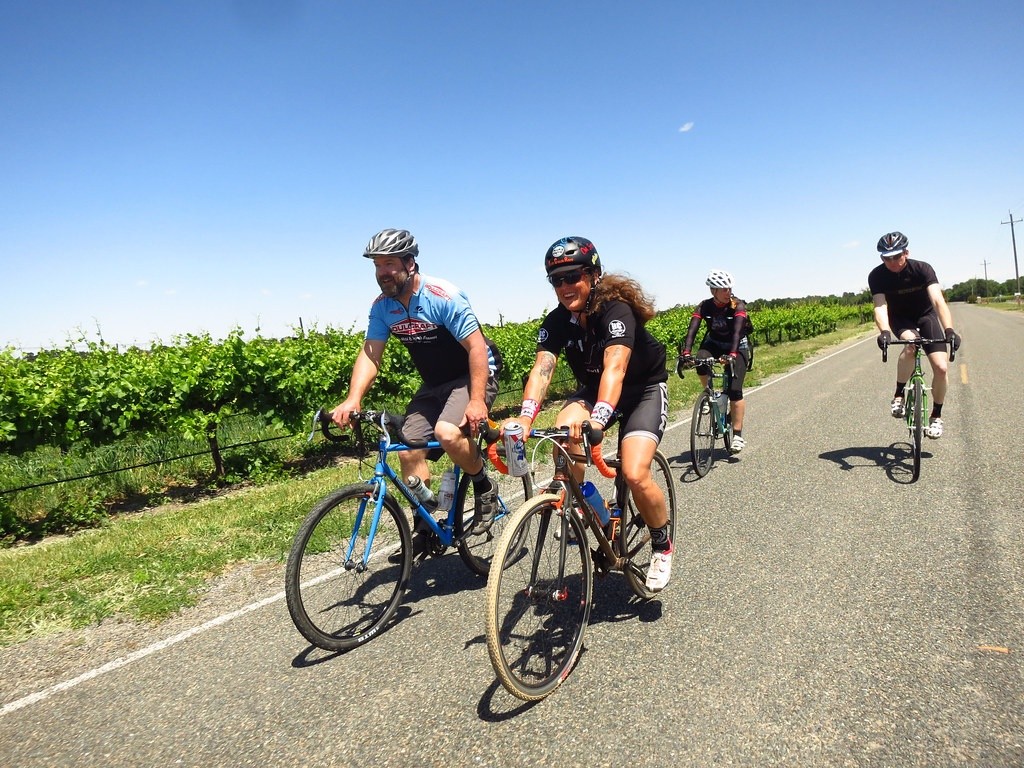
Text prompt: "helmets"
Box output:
[[363, 229, 419, 257], [877, 231, 910, 257], [705, 272, 732, 289], [545, 237, 602, 278]]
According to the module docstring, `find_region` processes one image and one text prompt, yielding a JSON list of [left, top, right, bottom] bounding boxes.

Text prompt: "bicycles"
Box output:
[[677, 354, 745, 478], [284, 406, 534, 650], [475, 415, 676, 699], [882, 326, 955, 477]]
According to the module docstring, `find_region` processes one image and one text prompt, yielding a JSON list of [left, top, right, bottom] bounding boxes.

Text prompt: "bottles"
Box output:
[[579, 480, 610, 525], [721, 390, 728, 410], [407, 475, 438, 513], [607, 499, 622, 539], [714, 391, 726, 413], [439, 466, 456, 511]]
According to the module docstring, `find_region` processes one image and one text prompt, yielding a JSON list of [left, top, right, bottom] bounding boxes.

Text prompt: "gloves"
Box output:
[[877, 330, 891, 350], [945, 328, 961, 351]]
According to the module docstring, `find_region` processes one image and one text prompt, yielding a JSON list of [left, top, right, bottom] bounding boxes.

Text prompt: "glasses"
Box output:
[[548, 271, 590, 288], [882, 251, 904, 261]]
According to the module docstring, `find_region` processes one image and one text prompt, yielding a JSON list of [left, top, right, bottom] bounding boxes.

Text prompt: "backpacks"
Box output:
[[705, 293, 754, 336]]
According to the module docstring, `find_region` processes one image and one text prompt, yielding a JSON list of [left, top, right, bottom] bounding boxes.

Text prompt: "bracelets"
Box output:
[[588, 401, 615, 428], [519, 399, 541, 423]]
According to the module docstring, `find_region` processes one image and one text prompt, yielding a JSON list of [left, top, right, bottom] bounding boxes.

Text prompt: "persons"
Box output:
[[497, 236, 673, 592], [868, 231, 961, 439], [329, 228, 500, 563], [682, 272, 750, 452]]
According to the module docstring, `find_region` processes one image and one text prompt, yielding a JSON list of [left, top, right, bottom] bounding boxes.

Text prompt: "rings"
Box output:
[[579, 435, 582, 436]]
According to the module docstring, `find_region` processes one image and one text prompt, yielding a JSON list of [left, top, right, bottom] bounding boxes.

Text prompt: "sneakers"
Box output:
[[471, 478, 499, 535], [891, 395, 905, 416], [554, 508, 589, 539], [731, 435, 744, 451], [388, 530, 431, 564], [646, 539, 674, 589], [698, 396, 715, 414], [928, 416, 943, 438]]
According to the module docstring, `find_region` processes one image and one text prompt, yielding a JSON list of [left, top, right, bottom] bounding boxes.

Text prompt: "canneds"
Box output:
[[504, 422, 529, 477]]
